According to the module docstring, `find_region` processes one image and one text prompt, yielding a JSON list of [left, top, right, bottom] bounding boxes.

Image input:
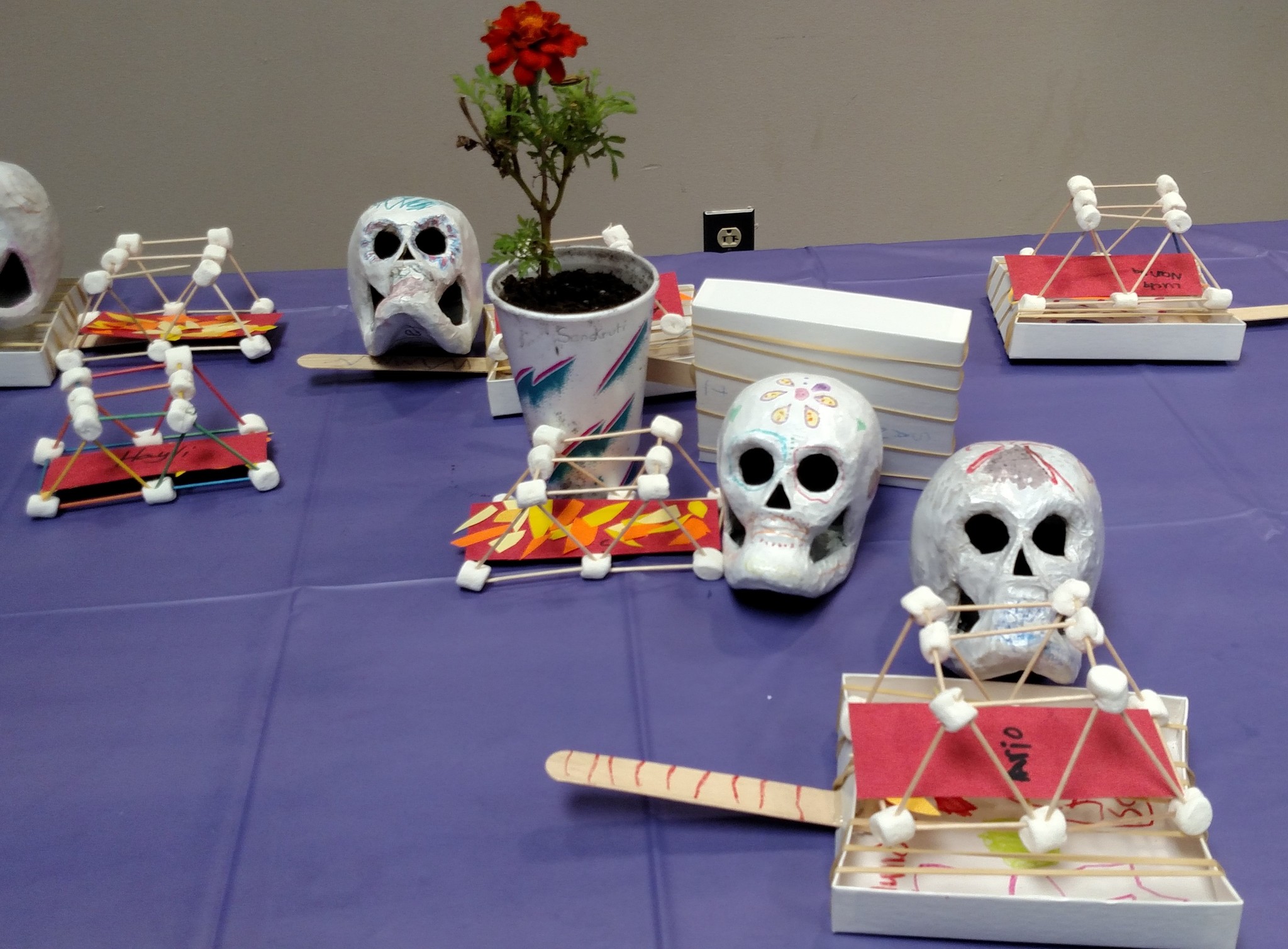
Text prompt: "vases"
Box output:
[[486, 245, 658, 501]]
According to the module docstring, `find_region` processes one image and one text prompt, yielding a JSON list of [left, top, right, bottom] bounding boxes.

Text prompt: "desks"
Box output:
[[0, 221, 1288, 949]]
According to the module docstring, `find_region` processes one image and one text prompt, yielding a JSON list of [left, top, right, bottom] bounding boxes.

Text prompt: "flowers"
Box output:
[[450, 0, 636, 287]]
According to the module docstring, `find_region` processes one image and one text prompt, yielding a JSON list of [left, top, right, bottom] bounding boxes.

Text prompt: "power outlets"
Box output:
[[704, 209, 755, 253]]
